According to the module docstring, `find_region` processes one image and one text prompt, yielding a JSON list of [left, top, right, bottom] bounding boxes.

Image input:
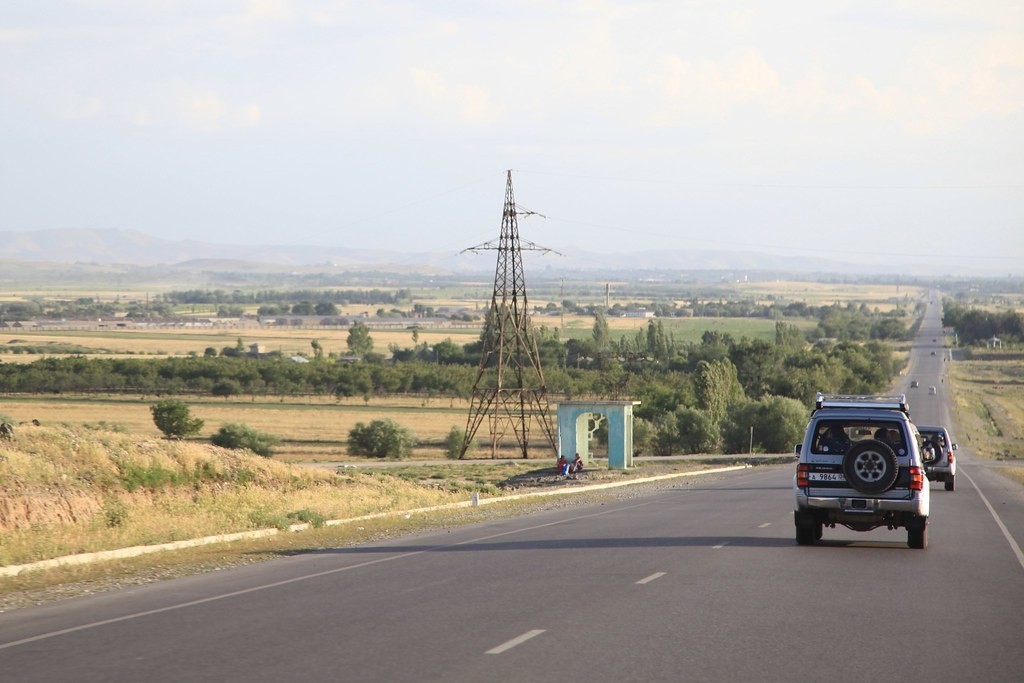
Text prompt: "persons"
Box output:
[[571, 453, 583, 472], [874, 428, 892, 444], [560, 455, 571, 475]]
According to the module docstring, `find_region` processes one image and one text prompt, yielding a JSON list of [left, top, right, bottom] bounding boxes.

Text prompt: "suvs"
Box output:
[[915, 425, 959, 491], [794, 391, 943, 551]]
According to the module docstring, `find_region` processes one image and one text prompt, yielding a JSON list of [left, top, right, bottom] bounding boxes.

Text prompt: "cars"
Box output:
[[287, 356, 311, 364]]
